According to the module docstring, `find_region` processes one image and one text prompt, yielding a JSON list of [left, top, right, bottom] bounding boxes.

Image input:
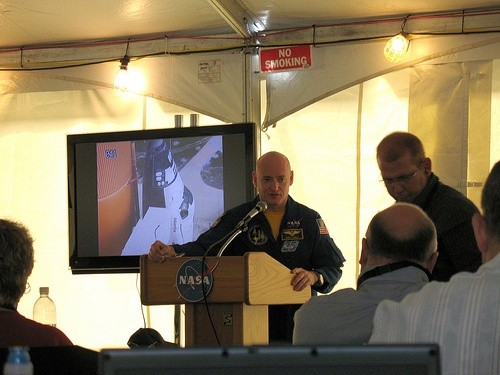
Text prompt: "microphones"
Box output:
[[235, 201, 268, 229]]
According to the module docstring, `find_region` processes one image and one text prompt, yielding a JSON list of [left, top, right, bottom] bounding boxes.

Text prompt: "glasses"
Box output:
[[380, 166, 421, 186]]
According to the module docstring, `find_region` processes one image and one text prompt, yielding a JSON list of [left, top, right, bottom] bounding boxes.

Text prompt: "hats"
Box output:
[[128, 328, 178, 350]]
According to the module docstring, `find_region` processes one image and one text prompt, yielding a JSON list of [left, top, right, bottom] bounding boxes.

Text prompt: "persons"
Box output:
[[376, 132, 483, 283], [369, 160, 500, 375], [0, 218, 74, 349], [147, 151, 346, 346], [292, 200, 438, 347]]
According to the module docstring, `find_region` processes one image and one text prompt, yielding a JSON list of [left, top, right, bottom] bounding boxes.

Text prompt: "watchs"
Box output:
[[311, 271, 325, 286]]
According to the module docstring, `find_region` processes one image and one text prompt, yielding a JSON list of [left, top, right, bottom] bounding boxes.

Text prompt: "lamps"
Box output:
[[383, 31, 415, 63]]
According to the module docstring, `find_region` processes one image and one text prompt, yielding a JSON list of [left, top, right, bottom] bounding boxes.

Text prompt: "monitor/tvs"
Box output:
[[67, 123, 256, 274]]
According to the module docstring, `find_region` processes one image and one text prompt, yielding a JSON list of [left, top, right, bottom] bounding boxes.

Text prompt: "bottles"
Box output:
[[3, 346, 33, 375], [32, 286, 56, 327]]
